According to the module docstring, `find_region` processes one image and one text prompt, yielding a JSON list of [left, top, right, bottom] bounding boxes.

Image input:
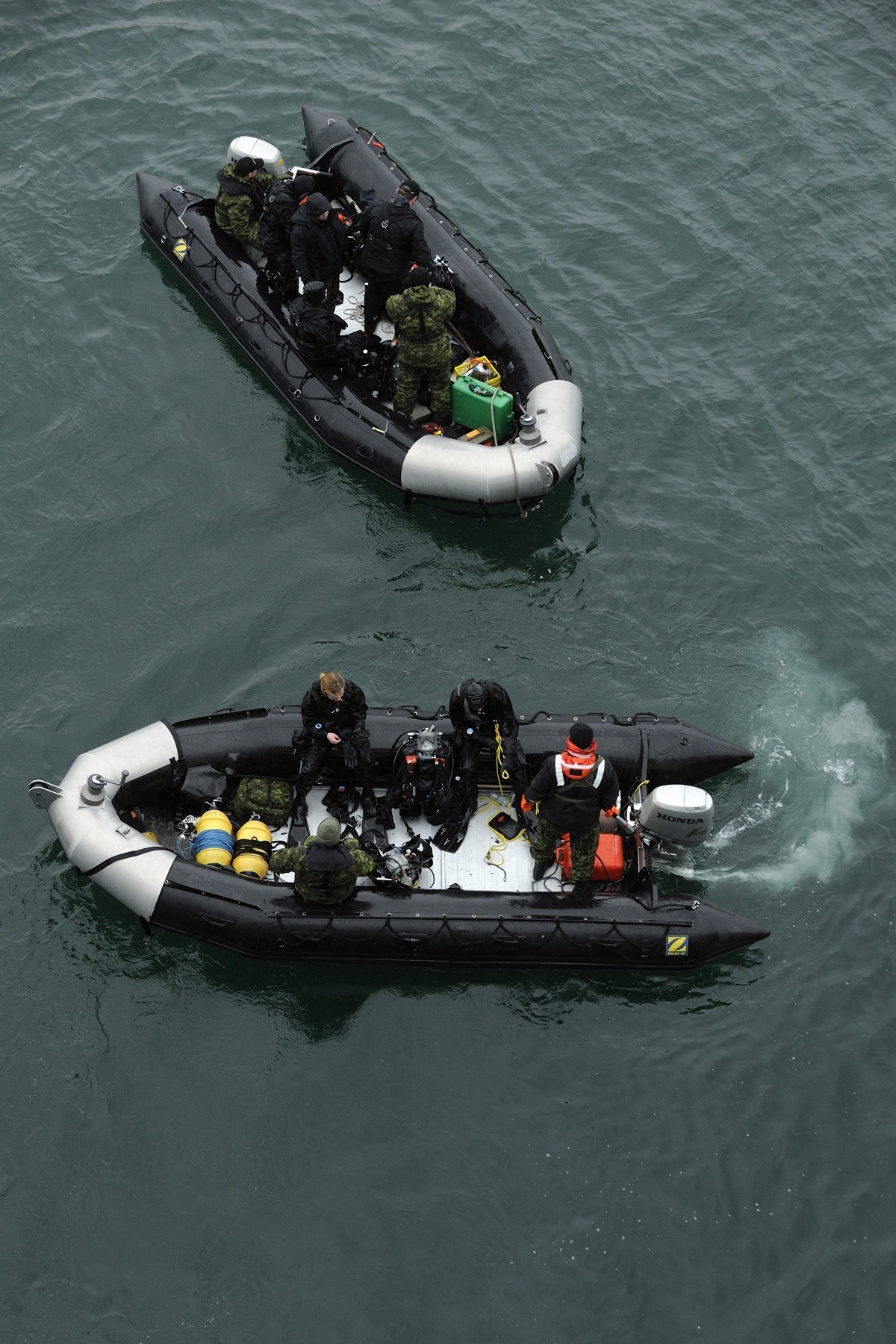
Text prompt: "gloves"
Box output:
[[343, 743, 359, 768], [481, 736, 498, 749], [500, 756, 514, 778]]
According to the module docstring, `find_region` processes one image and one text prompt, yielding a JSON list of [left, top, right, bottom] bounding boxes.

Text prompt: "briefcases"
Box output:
[[451, 375, 513, 440]]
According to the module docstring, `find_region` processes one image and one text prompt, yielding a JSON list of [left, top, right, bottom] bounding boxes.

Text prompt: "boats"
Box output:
[[134, 105, 583, 523], [28, 705, 770, 973]]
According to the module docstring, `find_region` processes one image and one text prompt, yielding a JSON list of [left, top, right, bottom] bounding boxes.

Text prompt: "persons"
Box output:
[[450, 679, 531, 824], [260, 175, 314, 274], [214, 157, 268, 252], [357, 180, 433, 333], [288, 673, 379, 818], [386, 267, 458, 426], [291, 192, 351, 315], [269, 817, 375, 906], [290, 278, 368, 369], [521, 723, 619, 899]]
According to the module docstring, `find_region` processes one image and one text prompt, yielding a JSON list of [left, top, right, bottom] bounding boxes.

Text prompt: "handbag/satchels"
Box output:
[[233, 778, 294, 828]]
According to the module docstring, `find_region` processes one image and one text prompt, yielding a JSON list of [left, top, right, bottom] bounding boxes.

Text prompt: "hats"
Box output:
[[466, 684, 486, 708], [304, 281, 326, 305], [317, 816, 341, 846], [234, 157, 264, 177], [402, 269, 431, 288], [569, 722, 594, 749], [400, 179, 421, 201], [292, 175, 313, 197]]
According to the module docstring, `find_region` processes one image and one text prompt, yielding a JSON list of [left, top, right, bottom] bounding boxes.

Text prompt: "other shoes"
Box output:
[[533, 851, 557, 881], [574, 881, 591, 897]]
